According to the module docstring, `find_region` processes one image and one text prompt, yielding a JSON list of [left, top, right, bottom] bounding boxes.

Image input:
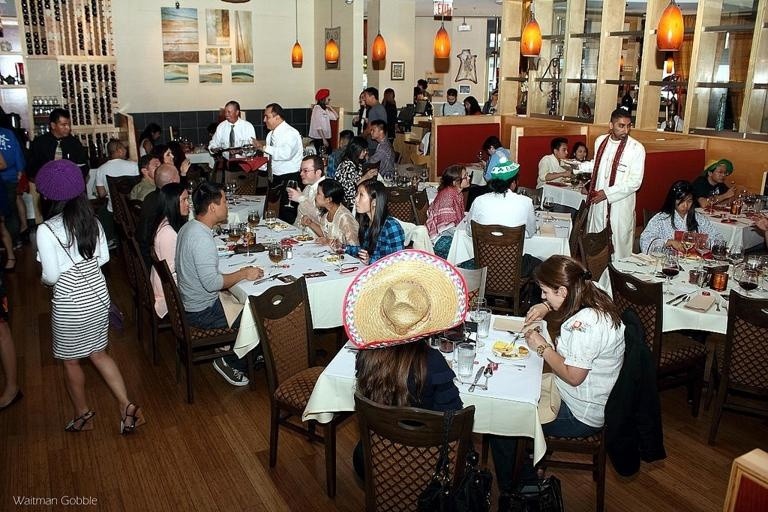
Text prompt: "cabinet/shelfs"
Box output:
[[1, 1, 34, 141]]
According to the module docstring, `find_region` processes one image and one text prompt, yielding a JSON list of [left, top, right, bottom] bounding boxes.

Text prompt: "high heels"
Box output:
[[66, 409, 95, 432], [19, 226, 31, 238], [120, 403, 145, 436]]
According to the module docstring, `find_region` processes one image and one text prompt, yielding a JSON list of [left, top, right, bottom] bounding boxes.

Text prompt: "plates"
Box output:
[[492, 342, 530, 360], [218, 259, 257, 276], [293, 235, 315, 243]]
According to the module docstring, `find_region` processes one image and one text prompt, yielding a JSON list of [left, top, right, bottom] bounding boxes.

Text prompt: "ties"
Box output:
[[230, 125, 235, 161], [267, 132, 273, 183], [54, 140, 62, 161]]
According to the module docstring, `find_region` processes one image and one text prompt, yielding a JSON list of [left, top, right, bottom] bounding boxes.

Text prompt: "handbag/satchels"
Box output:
[[21, 192, 36, 219], [108, 301, 126, 331], [417, 472, 493, 512], [510, 474, 564, 512], [418, 131, 430, 157]]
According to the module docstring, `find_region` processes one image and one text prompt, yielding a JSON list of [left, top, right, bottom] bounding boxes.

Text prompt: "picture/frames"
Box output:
[[389, 61, 406, 81], [458, 83, 472, 95]]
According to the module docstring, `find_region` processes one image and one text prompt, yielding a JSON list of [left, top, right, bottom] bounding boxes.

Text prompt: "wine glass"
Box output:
[[696, 238, 711, 263], [284, 179, 299, 209], [738, 270, 759, 297], [712, 240, 728, 265], [543, 197, 554, 217], [224, 181, 240, 209], [531, 195, 540, 211], [477, 151, 485, 165], [248, 210, 261, 235], [240, 140, 251, 161], [726, 245, 745, 277], [707, 194, 720, 215], [333, 236, 348, 272], [747, 255, 762, 272], [391, 167, 429, 188], [197, 140, 205, 152], [663, 258, 680, 296], [269, 246, 283, 274], [681, 231, 696, 265], [228, 223, 242, 246], [250, 142, 257, 162], [264, 210, 277, 240]]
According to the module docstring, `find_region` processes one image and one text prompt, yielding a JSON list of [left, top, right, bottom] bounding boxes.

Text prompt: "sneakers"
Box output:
[[215, 345, 231, 353], [212, 356, 249, 388]]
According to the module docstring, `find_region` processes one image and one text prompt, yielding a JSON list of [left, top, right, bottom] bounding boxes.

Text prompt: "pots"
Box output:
[[7, 113, 21, 129]]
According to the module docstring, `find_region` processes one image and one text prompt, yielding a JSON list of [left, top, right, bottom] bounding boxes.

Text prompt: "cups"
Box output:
[[473, 297, 487, 309], [470, 313, 485, 347], [711, 270, 729, 291], [476, 308, 493, 337], [690, 270, 700, 284], [457, 343, 476, 377]]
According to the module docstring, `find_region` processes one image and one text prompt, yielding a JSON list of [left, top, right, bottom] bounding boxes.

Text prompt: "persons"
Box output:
[[487, 253, 629, 510], [341, 249, 487, 511], [660, 103, 684, 133], [570, 110, 648, 263], [35, 159, 145, 438], [692, 158, 739, 209], [1, 80, 599, 390], [750, 211, 768, 250], [638, 179, 719, 255], [1, 267, 27, 414]]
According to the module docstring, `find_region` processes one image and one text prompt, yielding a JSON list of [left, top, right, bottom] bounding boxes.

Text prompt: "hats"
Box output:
[[315, 89, 329, 101], [350, 136, 368, 148], [702, 159, 734, 176], [36, 159, 85, 200], [491, 156, 520, 180], [447, 89, 457, 95], [343, 249, 469, 349], [492, 88, 498, 95]]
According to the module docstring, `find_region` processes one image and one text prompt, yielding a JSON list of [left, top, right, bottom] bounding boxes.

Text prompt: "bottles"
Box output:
[[32, 93, 60, 114], [729, 196, 764, 218]]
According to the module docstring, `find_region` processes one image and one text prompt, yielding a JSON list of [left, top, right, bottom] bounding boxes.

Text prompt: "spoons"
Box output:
[[483, 366, 494, 390], [673, 295, 690, 307]]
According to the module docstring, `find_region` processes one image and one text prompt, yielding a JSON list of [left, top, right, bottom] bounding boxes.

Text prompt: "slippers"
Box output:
[[0, 386, 24, 408]]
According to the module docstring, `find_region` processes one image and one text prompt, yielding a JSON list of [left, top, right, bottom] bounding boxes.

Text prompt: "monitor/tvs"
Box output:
[[396, 105, 415, 124], [415, 99, 428, 113]]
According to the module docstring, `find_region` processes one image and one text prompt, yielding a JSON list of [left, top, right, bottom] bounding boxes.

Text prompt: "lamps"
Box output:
[[666, 57, 674, 74], [654, 0, 686, 53], [519, 0, 543, 60], [432, 0, 452, 60], [291, 0, 386, 71]]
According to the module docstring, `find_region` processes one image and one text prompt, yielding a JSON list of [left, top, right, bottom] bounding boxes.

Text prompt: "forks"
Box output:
[[227, 258, 258, 266]]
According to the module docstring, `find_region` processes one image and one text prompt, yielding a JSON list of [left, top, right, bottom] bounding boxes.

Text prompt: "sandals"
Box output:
[[4, 257, 16, 272]]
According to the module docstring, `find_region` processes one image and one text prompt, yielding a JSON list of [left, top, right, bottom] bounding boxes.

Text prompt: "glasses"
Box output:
[[299, 168, 314, 173], [714, 169, 729, 175], [457, 172, 468, 182], [449, 94, 454, 95], [110, 136, 119, 151]]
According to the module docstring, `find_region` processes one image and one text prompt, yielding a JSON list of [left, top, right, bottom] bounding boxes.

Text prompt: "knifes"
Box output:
[[468, 365, 485, 394], [666, 293, 687, 305]]
